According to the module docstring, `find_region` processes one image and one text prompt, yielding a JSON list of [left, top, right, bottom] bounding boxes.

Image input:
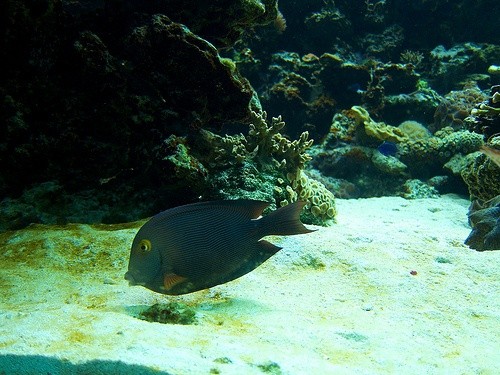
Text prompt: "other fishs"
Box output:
[[485, 91, 500, 103], [239, 0, 286, 35], [378, 138, 403, 156], [125, 190, 321, 297], [214, 51, 237, 70], [479, 143, 500, 168]]
